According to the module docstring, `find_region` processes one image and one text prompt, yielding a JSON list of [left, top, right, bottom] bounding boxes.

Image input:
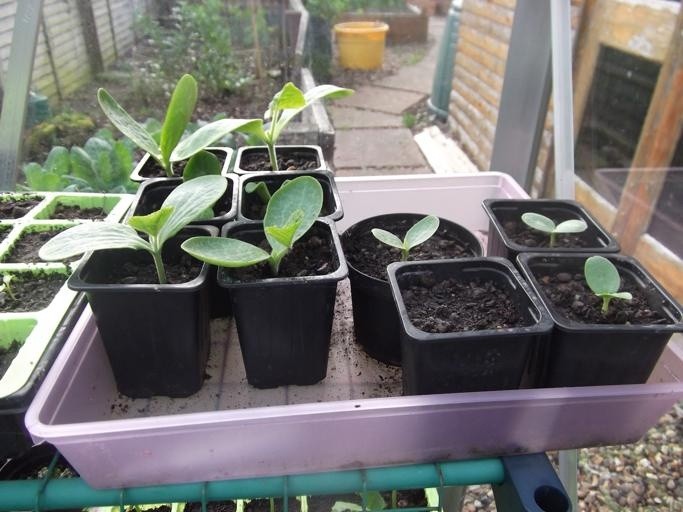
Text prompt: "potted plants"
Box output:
[[515, 251, 682, 387], [45, 70, 356, 397], [339, 210, 485, 366], [480, 197, 622, 260]]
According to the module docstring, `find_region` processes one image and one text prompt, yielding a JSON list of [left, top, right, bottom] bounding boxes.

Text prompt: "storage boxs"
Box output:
[[20, 165, 683, 497]]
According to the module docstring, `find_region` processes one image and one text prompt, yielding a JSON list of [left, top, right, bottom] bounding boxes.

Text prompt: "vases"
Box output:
[[387, 252, 553, 395]]
